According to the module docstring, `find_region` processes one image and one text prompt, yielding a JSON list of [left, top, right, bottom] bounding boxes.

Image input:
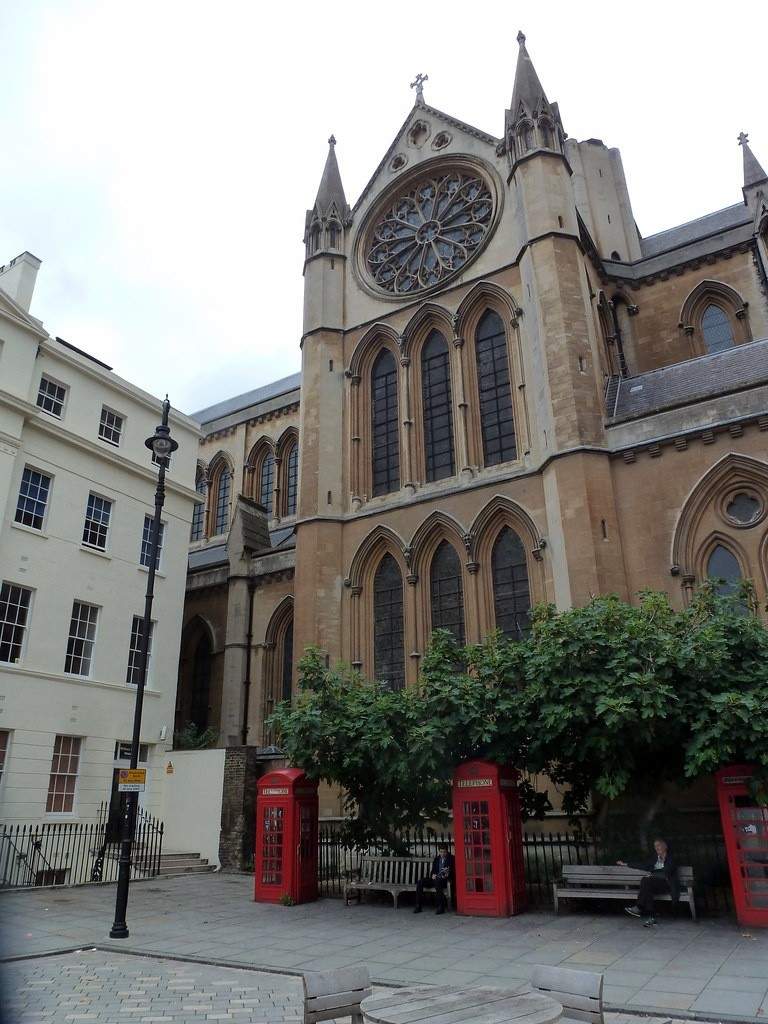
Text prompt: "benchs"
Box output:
[[551, 864, 697, 918], [342, 856, 455, 912]]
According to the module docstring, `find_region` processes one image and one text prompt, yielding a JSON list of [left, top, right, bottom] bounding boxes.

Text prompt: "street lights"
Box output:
[[109, 392, 180, 939]]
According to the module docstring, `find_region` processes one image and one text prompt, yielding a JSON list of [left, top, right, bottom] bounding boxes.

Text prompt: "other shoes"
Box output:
[[413, 904, 422, 914], [625, 906, 641, 918], [435, 905, 444, 915], [643, 917, 659, 927]]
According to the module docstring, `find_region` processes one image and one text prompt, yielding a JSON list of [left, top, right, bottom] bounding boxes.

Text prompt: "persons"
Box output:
[[413, 845, 454, 914], [617, 839, 675, 927]]
